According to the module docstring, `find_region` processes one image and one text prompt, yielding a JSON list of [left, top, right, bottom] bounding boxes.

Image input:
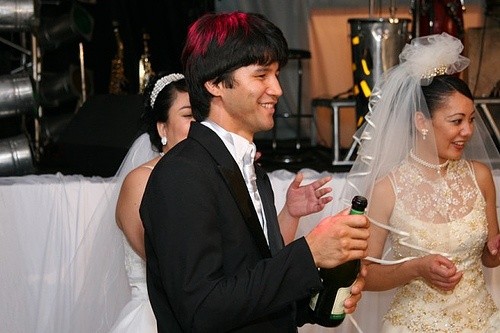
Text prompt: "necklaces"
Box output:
[[409, 148, 450, 173]]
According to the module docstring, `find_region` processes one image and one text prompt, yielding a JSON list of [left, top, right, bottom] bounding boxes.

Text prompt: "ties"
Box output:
[[254, 156, 285, 260]]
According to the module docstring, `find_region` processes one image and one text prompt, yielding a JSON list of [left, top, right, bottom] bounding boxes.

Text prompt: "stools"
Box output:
[[258, 48, 312, 164]]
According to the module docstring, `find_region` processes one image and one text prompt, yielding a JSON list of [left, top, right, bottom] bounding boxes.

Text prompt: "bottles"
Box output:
[[308, 195, 368, 328]]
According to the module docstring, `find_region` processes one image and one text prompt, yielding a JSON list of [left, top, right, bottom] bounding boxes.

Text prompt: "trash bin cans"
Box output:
[[348, 18, 411, 130]]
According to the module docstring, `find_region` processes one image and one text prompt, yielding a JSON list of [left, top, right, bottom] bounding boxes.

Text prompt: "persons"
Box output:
[[110, 11, 369, 333], [338, 34, 500, 333]]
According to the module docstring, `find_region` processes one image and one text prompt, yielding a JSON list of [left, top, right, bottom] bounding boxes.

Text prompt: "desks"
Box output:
[[311, 94, 500, 167]]
